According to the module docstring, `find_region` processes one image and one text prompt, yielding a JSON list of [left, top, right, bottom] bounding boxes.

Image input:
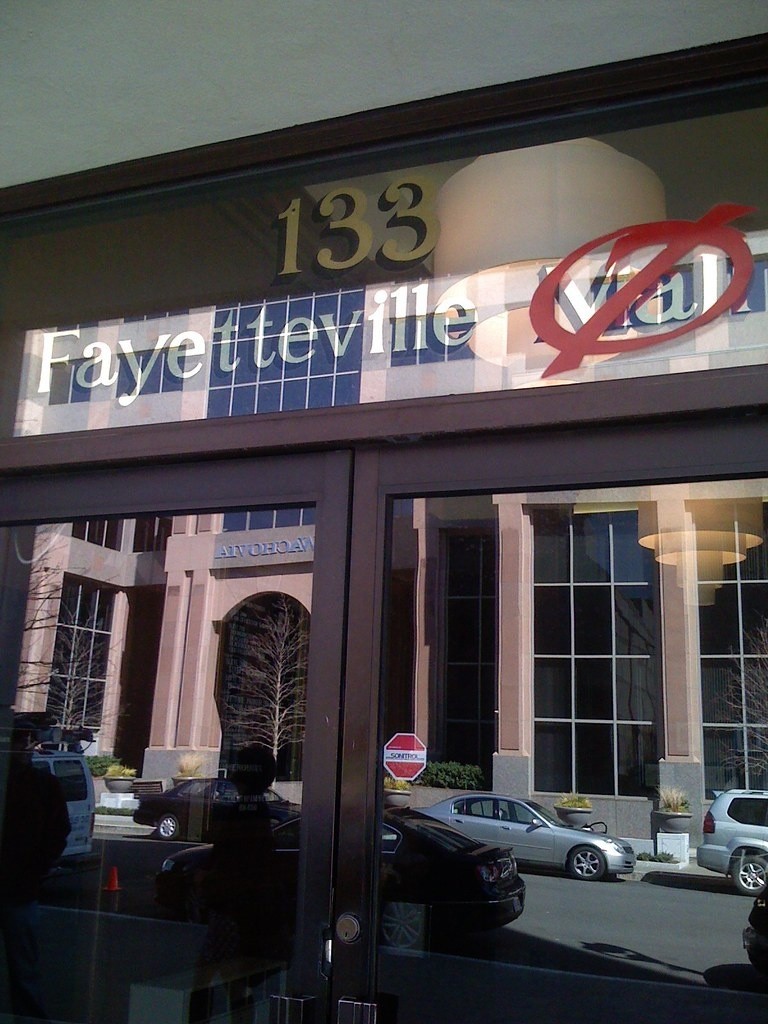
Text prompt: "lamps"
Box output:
[[431, 136, 669, 391]]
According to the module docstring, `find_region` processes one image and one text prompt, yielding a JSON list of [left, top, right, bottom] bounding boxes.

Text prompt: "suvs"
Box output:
[[0, 711, 100, 857], [697, 789, 768, 898]]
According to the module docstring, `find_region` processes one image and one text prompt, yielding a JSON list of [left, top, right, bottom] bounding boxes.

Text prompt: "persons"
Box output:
[[0, 723, 72, 1024], [188, 744, 276, 1024]]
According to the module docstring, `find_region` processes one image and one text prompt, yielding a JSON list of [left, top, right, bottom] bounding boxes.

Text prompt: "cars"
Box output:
[[134, 777, 301, 845], [151, 805, 528, 955], [412, 792, 637, 881]]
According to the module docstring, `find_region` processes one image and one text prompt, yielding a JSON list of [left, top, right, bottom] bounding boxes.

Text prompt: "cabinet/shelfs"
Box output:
[[127, 957, 287, 1024]]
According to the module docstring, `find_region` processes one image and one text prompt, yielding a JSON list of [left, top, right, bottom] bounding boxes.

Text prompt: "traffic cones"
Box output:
[[102, 866, 123, 893]]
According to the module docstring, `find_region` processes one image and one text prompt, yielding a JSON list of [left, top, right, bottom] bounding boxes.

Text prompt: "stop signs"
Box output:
[[382, 734, 428, 782]]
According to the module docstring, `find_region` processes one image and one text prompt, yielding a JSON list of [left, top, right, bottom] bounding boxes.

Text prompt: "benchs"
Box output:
[[132, 780, 163, 799]]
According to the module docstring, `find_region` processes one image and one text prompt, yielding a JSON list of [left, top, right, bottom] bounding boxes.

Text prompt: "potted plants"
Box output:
[[171, 753, 207, 787], [653, 784, 693, 833], [102, 765, 137, 793], [382, 776, 412, 807], [553, 791, 593, 828]]
